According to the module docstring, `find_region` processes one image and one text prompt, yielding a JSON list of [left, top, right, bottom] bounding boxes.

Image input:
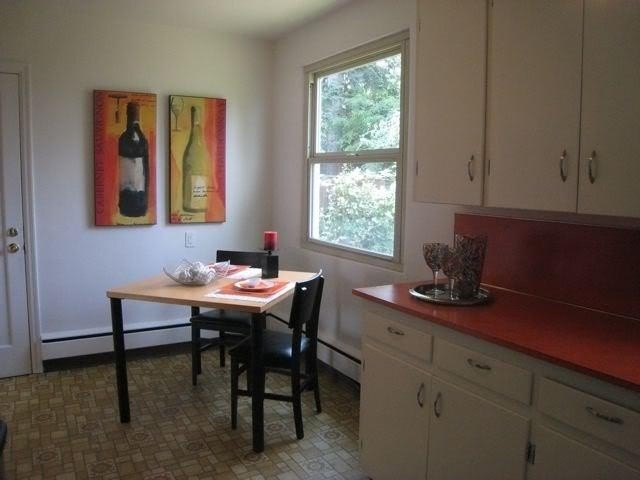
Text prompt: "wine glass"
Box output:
[[422, 243, 449, 295], [438, 246, 465, 301], [171, 96, 184, 131]]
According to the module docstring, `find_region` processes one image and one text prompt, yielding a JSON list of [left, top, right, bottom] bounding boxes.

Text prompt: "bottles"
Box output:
[[181, 106, 212, 212], [116, 102, 149, 217]]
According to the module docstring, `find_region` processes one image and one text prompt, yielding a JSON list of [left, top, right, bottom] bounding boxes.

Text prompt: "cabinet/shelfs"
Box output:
[[359, 301, 534, 480], [486, 0, 640, 218], [414, 0, 487, 206], [533, 375, 640, 480]]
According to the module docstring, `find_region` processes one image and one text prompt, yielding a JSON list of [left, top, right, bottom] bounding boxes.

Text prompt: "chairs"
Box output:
[[190, 250, 268, 386], [226, 269, 321, 439]]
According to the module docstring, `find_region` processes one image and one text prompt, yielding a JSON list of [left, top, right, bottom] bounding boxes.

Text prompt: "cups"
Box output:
[[454, 233, 488, 298]]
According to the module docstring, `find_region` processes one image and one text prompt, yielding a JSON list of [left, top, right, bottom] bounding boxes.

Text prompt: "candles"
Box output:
[[264, 231, 277, 250]]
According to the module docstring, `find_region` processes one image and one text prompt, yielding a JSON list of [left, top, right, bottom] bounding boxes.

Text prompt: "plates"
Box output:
[[234, 279, 278, 292]]
